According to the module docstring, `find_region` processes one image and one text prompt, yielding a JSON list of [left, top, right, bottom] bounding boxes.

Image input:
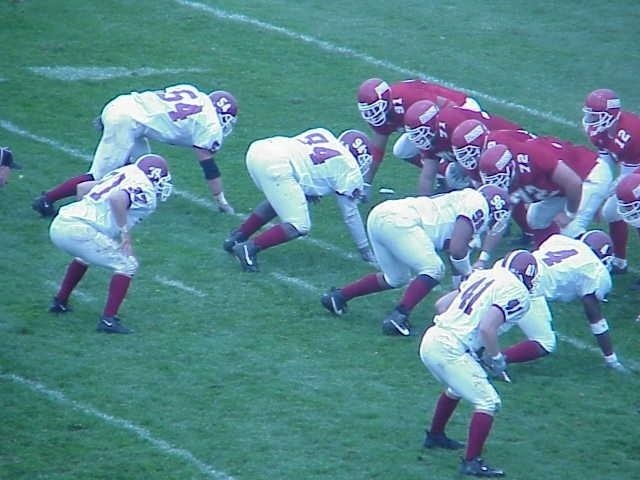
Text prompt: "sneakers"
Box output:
[[223, 233, 260, 272], [507, 233, 535, 246], [480, 352, 512, 383], [424, 429, 466, 450], [460, 455, 504, 476], [320, 291, 346, 315], [47, 299, 71, 313], [382, 319, 421, 336], [213, 192, 235, 215], [600, 256, 630, 274], [31, 193, 56, 215], [97, 317, 136, 333]]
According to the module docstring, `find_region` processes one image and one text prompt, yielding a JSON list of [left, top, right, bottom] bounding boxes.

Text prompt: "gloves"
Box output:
[[94, 115, 104, 131], [605, 353, 634, 374]]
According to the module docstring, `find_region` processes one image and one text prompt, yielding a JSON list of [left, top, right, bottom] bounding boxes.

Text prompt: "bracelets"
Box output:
[[118, 223, 128, 234], [603, 352, 618, 364], [212, 191, 227, 206], [491, 352, 503, 361]]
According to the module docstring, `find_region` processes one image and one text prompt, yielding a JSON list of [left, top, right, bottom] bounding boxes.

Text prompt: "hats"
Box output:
[[0, 146, 23, 170]]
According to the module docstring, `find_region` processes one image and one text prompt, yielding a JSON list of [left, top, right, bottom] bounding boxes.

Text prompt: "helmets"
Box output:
[[501, 250, 540, 296], [582, 89, 622, 137], [135, 154, 172, 201], [477, 183, 512, 237], [338, 130, 374, 177], [357, 78, 517, 193], [208, 90, 238, 135], [615, 173, 640, 229], [580, 230, 616, 265]]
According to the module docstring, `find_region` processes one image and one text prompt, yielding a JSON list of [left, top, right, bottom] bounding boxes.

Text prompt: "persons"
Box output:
[[46, 154, 173, 334], [404, 89, 639, 321], [31, 84, 239, 217], [321, 184, 511, 337], [423, 249, 538, 477], [356, 77, 482, 203], [483, 229, 625, 378], [0, 147, 23, 188], [222, 127, 380, 273]]
